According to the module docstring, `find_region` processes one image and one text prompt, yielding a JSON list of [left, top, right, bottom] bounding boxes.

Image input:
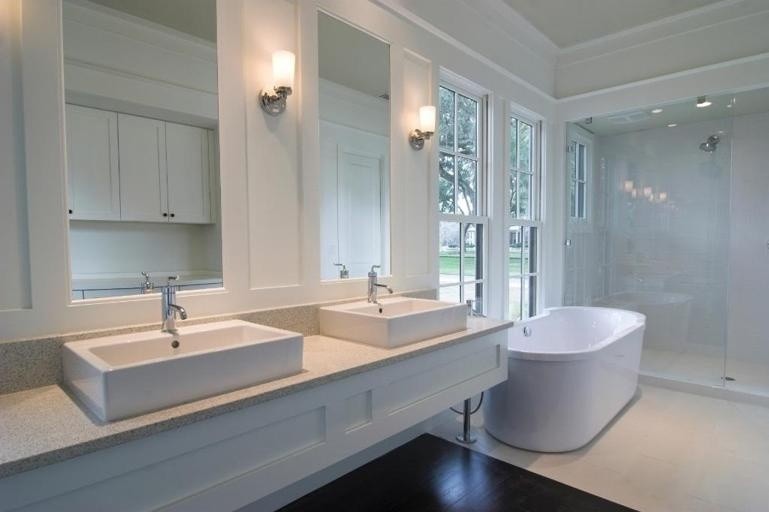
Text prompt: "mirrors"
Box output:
[[60, 0, 224, 303], [315, 6, 393, 284]]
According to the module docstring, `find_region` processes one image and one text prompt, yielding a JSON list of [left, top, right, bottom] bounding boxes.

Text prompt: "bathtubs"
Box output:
[[484, 304, 646, 453]]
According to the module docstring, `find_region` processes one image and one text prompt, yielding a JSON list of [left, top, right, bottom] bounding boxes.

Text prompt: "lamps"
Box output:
[[408, 105, 436, 152], [695, 96, 711, 111], [258, 49, 296, 117]]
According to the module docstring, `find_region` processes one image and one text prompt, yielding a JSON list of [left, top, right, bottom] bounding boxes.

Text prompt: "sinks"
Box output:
[[319, 298, 468, 347], [62, 320, 304, 421]]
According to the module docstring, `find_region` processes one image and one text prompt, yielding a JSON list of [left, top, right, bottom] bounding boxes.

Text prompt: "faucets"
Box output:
[[140, 269, 152, 292], [375, 282, 393, 295], [332, 262, 350, 279]]
